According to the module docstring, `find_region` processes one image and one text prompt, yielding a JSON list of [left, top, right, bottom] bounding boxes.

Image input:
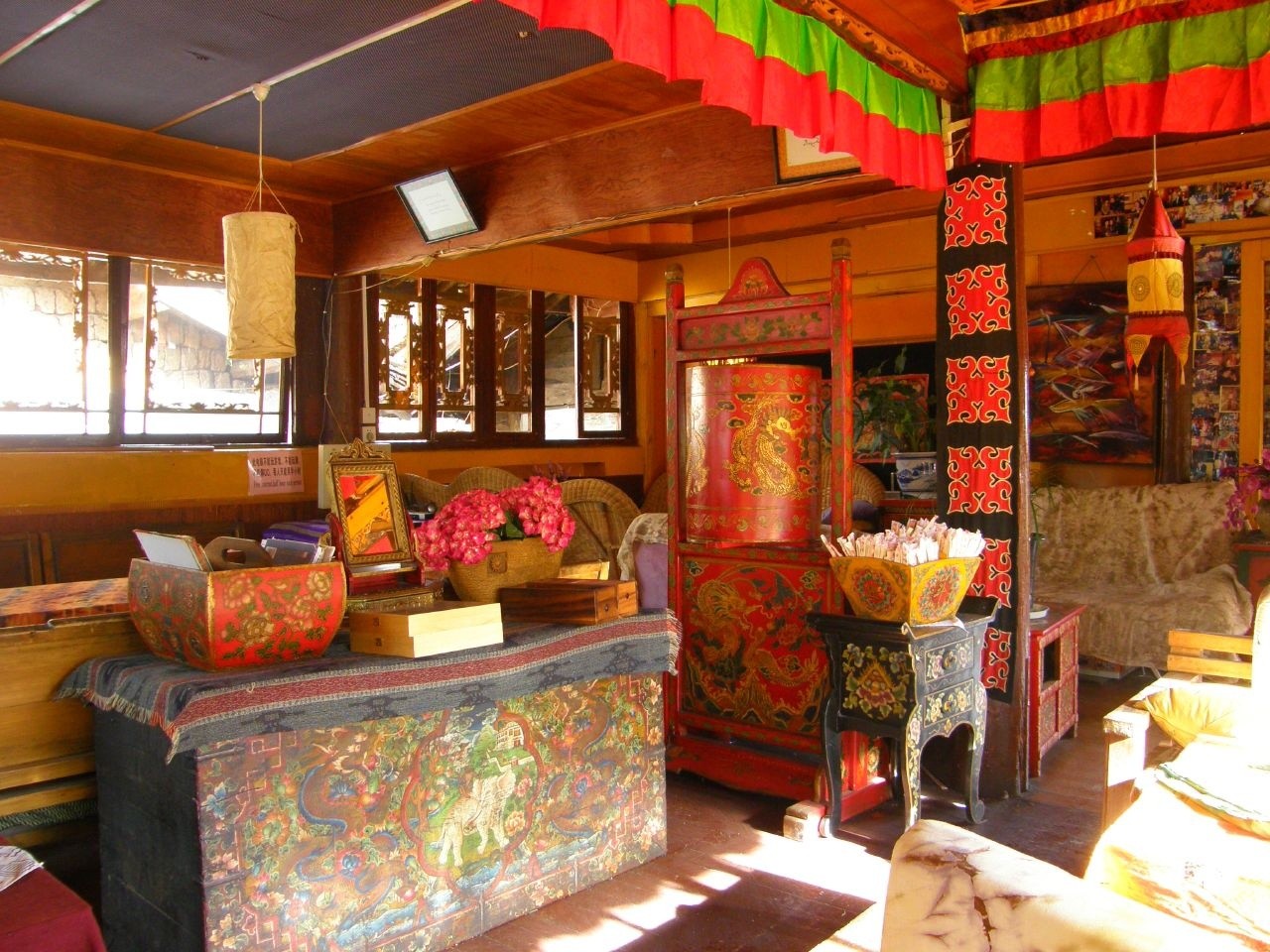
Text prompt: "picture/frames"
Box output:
[[394, 169, 480, 244], [772, 124, 866, 185], [327, 438, 413, 568]]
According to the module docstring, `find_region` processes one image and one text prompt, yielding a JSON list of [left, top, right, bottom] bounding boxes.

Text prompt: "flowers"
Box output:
[[416, 474, 572, 568], [1222, 448, 1270, 535]]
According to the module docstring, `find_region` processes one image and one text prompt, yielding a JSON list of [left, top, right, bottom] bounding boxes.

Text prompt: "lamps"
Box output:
[[1127, 135, 1190, 389], [219, 83, 304, 358]]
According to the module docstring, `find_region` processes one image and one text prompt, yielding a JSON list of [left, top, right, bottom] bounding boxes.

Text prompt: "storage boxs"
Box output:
[[128, 559, 638, 670]]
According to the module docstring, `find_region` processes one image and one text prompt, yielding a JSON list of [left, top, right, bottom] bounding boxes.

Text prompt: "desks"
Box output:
[[804, 596, 1000, 831], [51, 605, 683, 951]]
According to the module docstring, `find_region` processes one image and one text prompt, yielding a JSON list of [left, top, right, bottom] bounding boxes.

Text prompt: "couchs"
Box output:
[[882, 630, 1270, 952]]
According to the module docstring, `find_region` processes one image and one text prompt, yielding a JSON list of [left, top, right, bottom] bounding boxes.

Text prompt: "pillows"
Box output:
[[1143, 680, 1265, 762]]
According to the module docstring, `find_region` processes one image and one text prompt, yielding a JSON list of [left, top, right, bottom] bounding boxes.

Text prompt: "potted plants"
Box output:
[[854, 348, 936, 499]]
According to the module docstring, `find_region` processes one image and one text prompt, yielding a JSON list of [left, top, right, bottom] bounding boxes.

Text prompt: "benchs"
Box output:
[[1023, 485, 1252, 681]]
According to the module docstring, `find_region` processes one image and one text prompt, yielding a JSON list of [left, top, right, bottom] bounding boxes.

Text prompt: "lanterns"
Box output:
[[222, 210, 296, 360], [1125, 190, 1191, 369]]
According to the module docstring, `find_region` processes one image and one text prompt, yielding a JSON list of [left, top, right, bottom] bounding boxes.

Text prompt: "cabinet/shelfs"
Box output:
[[1027, 604, 1085, 776]]
